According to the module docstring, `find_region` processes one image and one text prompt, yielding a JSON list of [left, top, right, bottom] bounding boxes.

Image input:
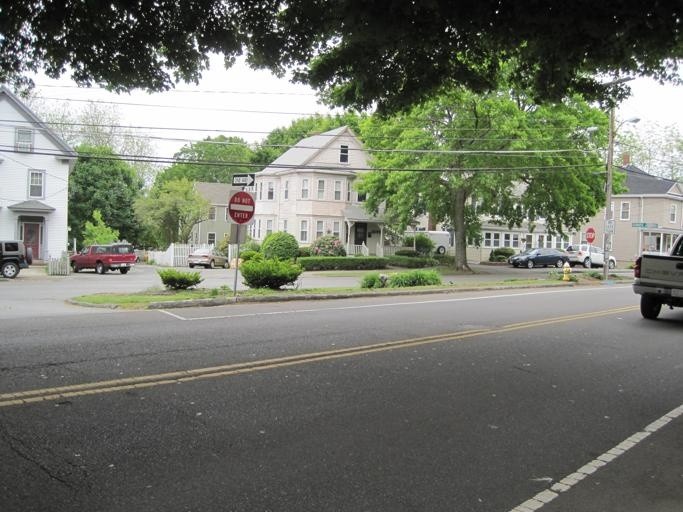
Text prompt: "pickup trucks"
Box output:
[[631, 233, 683, 320]]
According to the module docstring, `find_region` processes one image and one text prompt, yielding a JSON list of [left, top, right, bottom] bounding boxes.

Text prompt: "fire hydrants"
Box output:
[[562, 261, 571, 280]]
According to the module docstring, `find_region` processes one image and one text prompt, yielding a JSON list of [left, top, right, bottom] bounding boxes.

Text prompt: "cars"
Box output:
[[506, 246, 570, 270], [187, 247, 229, 270]]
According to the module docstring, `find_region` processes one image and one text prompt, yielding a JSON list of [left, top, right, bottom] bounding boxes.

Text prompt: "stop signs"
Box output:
[[227, 191, 256, 224]]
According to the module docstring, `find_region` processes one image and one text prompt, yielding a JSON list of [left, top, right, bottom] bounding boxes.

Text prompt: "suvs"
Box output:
[[566, 244, 617, 268], [68, 243, 136, 275], [0, 239, 33, 279]]
